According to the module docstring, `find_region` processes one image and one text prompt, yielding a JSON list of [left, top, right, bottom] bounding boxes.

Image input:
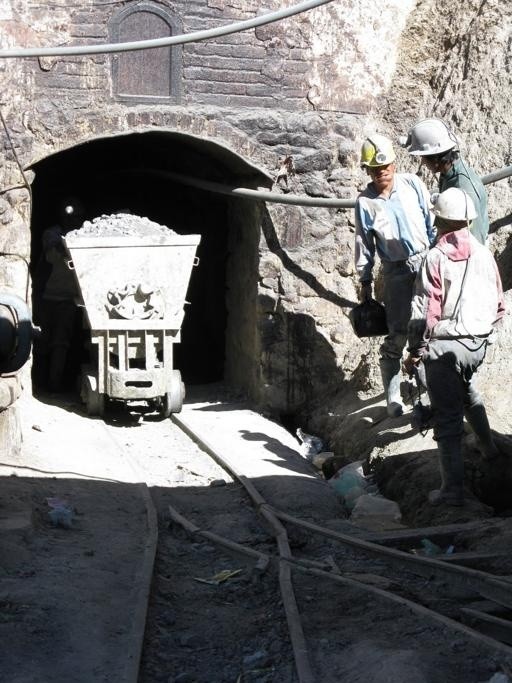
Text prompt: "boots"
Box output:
[[463, 405, 497, 461], [411, 359, 428, 390], [426, 429, 467, 508], [379, 354, 407, 418]]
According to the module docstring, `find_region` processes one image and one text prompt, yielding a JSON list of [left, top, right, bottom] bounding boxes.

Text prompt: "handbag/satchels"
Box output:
[[347, 297, 390, 339]]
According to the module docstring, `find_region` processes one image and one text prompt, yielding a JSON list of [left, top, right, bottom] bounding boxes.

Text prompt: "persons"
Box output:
[[397, 184, 506, 509], [351, 134, 439, 420], [404, 116, 490, 252]]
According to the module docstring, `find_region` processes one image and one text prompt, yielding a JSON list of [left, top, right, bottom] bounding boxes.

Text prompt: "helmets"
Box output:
[[427, 185, 480, 221], [357, 136, 397, 168], [405, 117, 457, 156]]
